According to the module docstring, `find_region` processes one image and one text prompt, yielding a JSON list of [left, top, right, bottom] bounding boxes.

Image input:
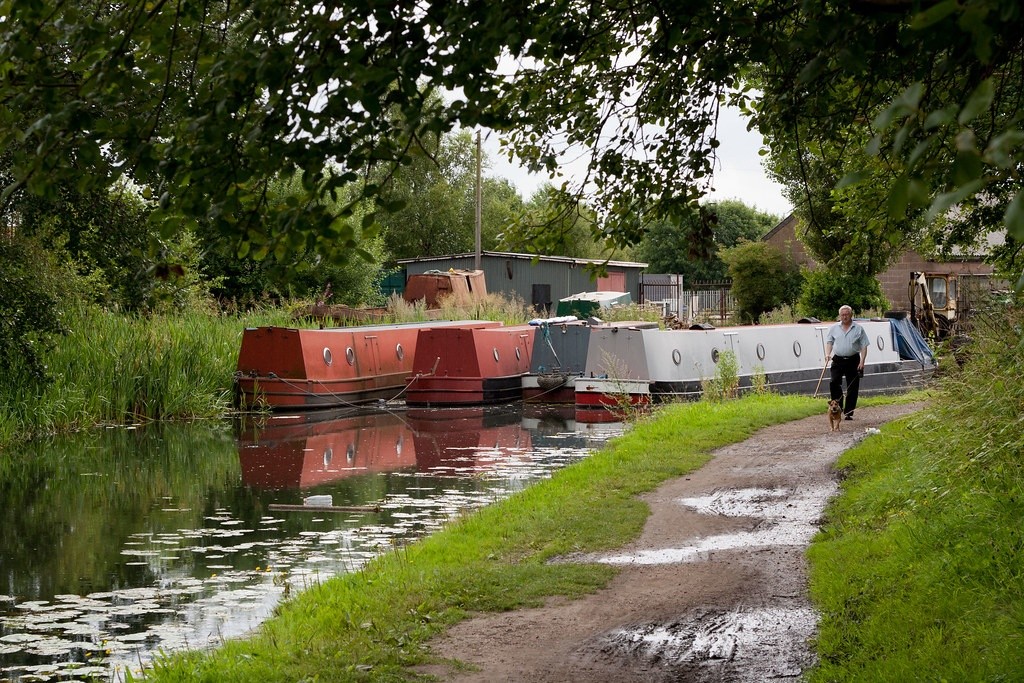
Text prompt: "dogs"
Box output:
[[827, 399, 842, 431]]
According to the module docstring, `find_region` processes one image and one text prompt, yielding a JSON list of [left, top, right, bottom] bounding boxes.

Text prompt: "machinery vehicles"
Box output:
[[908, 270, 960, 348]]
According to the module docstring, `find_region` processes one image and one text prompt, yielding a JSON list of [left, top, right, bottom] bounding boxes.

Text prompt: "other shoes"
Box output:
[[844, 414, 852, 420]]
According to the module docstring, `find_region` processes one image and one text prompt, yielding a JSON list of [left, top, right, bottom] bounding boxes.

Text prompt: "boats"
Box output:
[[520, 314, 937, 422], [233, 322, 506, 412], [404, 316, 535, 407]]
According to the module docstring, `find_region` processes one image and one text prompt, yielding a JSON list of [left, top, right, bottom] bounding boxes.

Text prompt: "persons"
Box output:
[[825, 305, 869, 421]]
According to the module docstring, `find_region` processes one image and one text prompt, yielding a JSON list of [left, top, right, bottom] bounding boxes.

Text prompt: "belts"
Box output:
[[835, 354, 858, 360]]
